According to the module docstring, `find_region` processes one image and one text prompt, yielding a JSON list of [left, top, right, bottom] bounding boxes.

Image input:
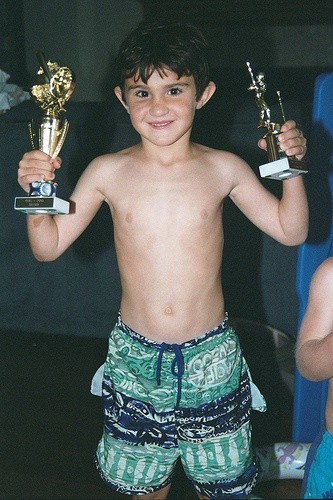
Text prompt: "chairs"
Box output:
[[291, 71, 333, 443]]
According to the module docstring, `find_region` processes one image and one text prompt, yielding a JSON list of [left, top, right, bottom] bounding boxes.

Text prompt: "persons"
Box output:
[[295, 256, 333, 500], [17, 20, 309, 500]]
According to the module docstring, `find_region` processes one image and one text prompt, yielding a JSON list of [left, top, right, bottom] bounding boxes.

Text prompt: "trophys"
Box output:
[[14, 51, 76, 214], [246, 61, 311, 181]]
